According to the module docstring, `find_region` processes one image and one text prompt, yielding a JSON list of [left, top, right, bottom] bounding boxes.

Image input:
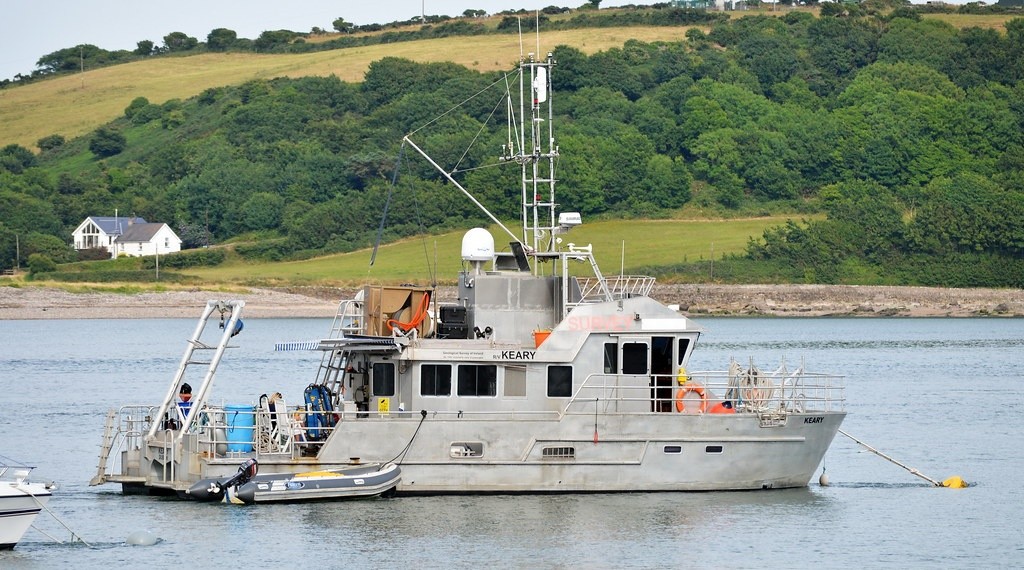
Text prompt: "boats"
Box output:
[[0, 452, 58, 551], [185, 458, 402, 505], [89, 2, 848, 497]]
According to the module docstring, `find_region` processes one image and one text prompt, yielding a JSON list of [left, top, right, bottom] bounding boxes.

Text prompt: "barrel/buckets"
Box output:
[[225, 404, 254, 453], [535, 331, 551, 349]]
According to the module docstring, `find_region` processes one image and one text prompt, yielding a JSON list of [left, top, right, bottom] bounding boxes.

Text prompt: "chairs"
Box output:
[[257, 396, 295, 453], [272, 398, 307, 456]]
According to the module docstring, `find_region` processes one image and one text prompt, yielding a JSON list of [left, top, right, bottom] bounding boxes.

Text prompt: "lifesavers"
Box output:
[[675, 383, 709, 414]]
